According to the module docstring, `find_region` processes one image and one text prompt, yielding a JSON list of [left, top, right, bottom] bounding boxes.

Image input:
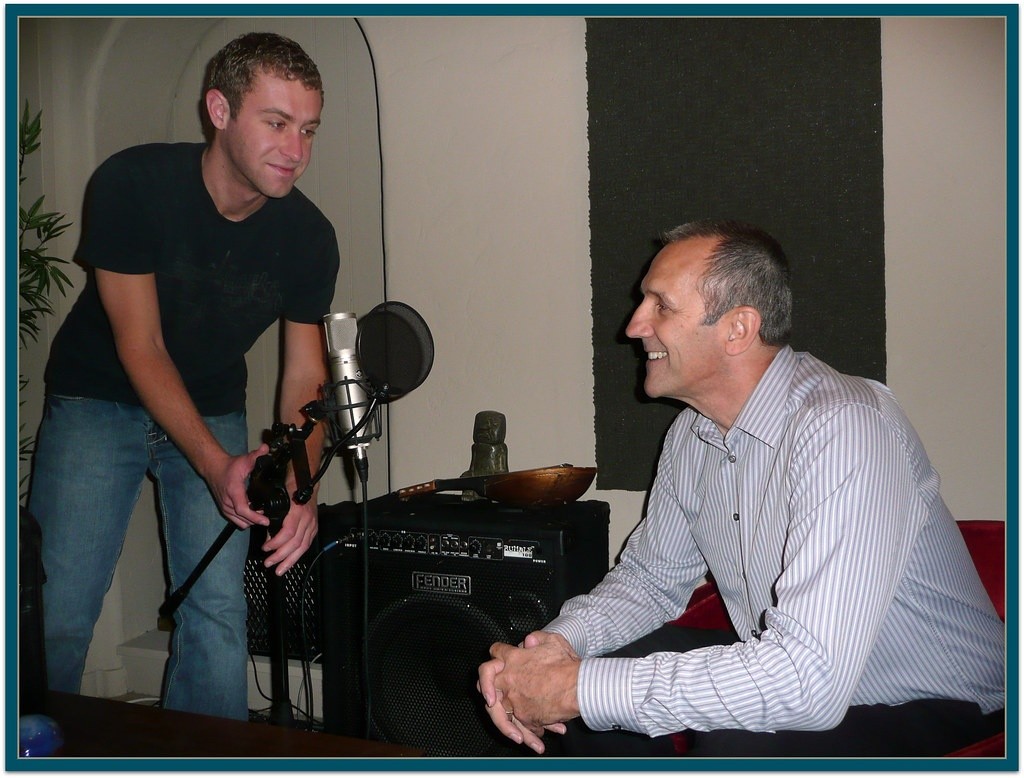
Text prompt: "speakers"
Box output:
[[321, 498, 612, 757]]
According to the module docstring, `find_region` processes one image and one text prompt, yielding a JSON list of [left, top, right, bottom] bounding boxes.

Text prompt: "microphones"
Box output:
[[324, 311, 374, 480]]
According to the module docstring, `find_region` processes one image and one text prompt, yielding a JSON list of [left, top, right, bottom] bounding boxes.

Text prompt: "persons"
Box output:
[[476, 211, 1007, 759], [28, 32, 341, 722]]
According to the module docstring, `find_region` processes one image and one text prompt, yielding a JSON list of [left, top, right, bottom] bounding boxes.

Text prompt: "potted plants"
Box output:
[[19, 96, 73, 705]]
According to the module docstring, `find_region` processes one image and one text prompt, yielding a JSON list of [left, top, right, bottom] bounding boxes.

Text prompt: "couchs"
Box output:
[[667, 520, 1005, 757]]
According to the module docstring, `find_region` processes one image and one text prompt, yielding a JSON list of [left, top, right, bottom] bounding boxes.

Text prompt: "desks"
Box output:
[[47, 687, 426, 756]]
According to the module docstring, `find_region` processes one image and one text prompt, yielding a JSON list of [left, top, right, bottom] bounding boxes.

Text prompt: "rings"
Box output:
[[505, 710, 514, 715]]
